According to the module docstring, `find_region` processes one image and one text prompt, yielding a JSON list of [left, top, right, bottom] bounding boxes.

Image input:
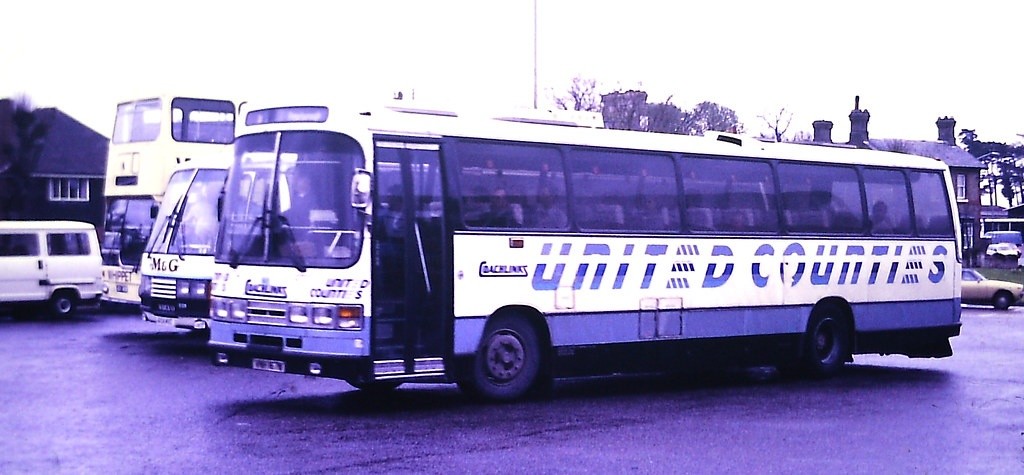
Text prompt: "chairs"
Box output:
[[487, 195, 954, 234]]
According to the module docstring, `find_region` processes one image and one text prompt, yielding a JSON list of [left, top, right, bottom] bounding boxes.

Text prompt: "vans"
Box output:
[[0, 220, 105, 317], [984, 231, 1022, 246]]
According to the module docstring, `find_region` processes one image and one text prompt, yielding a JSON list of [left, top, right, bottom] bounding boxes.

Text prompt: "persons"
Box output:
[[638, 192, 671, 229], [870, 199, 895, 233], [484, 187, 522, 226], [532, 181, 568, 227]]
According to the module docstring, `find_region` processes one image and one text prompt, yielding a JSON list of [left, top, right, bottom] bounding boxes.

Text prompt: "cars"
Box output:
[[987, 244, 1017, 259], [961, 269, 1024, 312]]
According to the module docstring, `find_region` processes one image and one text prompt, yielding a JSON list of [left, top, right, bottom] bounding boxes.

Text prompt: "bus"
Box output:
[[139, 154, 235, 332], [207, 94, 965, 407], [97, 92, 237, 308]]
